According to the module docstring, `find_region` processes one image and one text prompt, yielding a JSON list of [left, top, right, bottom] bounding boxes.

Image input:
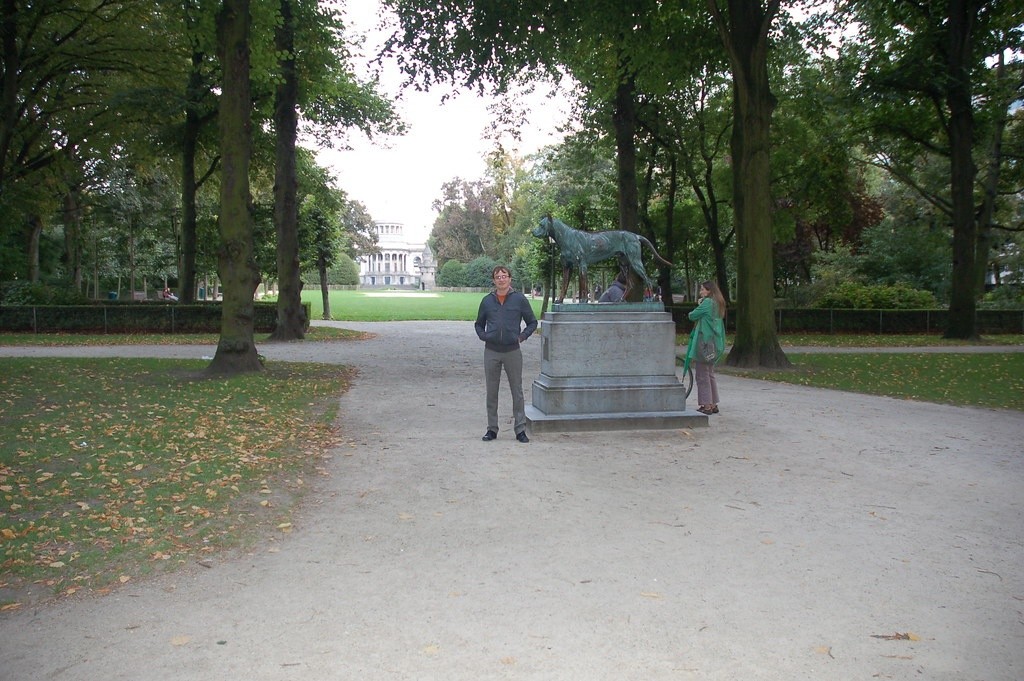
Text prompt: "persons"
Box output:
[[684, 281, 726, 414], [597, 270, 627, 302], [475, 266, 538, 443], [163, 288, 179, 302]]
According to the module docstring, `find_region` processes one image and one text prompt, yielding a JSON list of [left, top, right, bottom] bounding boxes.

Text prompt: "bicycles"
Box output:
[[674, 357, 694, 399]]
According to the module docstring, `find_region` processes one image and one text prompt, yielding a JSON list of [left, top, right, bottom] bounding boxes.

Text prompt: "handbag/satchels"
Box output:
[[695, 331, 717, 363]]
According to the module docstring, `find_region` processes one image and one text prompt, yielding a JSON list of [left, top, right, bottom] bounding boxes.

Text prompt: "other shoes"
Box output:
[[710, 403, 719, 413], [697, 406, 712, 415]]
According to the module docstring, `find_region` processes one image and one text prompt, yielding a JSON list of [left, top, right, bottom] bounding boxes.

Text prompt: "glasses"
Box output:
[[494, 274, 510, 279]]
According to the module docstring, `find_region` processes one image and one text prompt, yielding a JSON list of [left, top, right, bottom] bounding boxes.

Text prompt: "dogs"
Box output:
[[531, 213, 673, 303]]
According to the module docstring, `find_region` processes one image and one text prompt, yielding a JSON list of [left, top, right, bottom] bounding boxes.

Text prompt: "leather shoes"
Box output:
[[516, 431, 529, 443], [482, 430, 497, 441]]
